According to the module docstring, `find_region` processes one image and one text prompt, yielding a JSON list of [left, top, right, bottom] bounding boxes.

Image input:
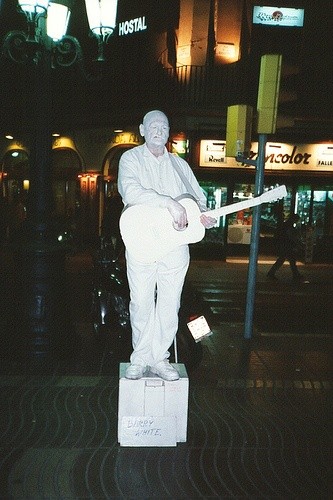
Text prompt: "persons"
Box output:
[[267, 214, 304, 280], [118, 110, 218, 381]]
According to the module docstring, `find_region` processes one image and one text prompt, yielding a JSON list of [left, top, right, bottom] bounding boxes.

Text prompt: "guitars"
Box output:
[[119, 184, 288, 265]]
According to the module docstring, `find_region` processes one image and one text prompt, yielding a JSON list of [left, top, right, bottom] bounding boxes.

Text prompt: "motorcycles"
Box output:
[[88, 234, 203, 371]]
